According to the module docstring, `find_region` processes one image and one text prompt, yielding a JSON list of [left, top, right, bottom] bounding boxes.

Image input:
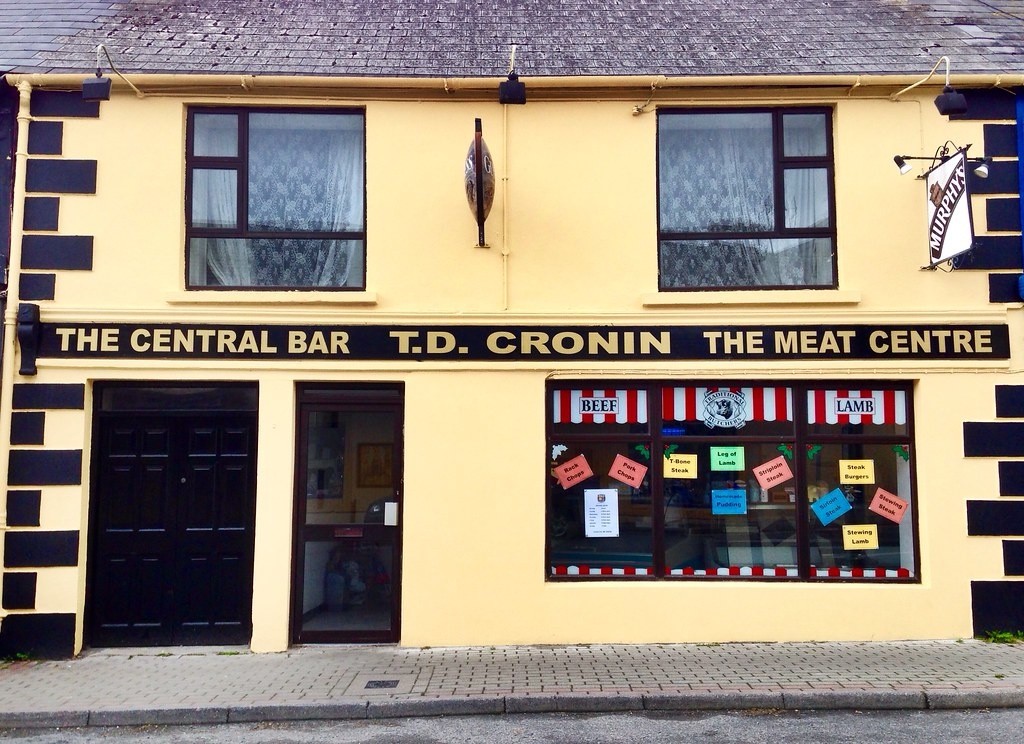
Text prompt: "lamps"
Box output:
[[895, 155, 942, 175], [890, 56, 969, 115], [499, 48, 527, 107], [968, 157, 992, 178], [84, 46, 145, 102]]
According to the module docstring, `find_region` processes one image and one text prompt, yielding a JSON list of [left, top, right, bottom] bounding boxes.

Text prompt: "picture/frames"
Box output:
[[358, 442, 393, 487]]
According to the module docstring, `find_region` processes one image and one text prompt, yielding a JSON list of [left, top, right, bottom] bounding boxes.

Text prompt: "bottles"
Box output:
[[749, 477, 760, 503]]
